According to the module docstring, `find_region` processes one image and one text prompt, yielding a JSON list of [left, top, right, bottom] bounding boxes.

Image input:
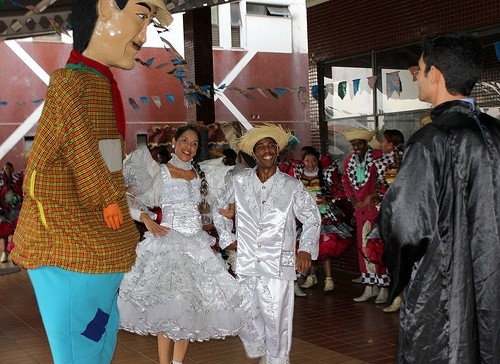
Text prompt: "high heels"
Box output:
[[382, 297, 402, 312], [374, 286, 388, 304], [352, 285, 379, 302], [294, 283, 306, 296]]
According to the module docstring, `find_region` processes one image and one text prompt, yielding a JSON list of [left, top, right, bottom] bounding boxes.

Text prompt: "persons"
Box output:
[[279, 127, 404, 311], [197, 148, 248, 277], [0, 161, 25, 263], [131, 125, 235, 364], [378, 34, 500, 364], [14, 0, 174, 364], [212, 133, 322, 364]]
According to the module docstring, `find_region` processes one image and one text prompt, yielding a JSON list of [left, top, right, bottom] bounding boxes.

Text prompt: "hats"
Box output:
[[340, 127, 376, 142], [235, 122, 291, 156]]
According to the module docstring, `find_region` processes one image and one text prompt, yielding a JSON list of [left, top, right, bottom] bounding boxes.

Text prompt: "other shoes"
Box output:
[[324, 277, 334, 290], [352, 277, 363, 283], [301, 275, 317, 288], [1, 251, 9, 262]]
